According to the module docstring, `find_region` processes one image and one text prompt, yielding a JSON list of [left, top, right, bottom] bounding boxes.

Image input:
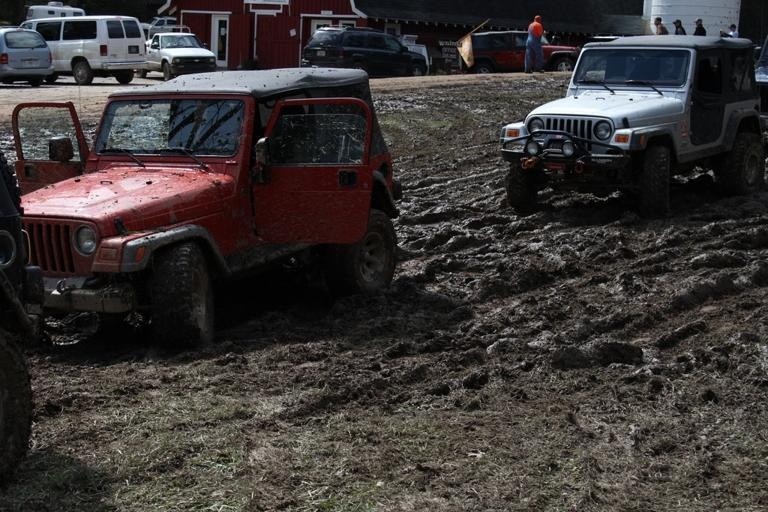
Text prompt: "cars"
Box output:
[[1, 1, 218, 84]]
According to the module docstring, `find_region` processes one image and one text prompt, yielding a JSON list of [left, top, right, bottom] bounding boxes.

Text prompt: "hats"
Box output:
[[694, 18, 702, 22], [672, 19, 681, 24]]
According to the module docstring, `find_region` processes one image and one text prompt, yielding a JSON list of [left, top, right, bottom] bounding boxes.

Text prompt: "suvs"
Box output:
[[498, 34, 764, 215], [457, 31, 580, 72], [301, 27, 429, 80], [11, 64, 403, 346], [1, 149, 46, 495], [752, 36, 768, 149]]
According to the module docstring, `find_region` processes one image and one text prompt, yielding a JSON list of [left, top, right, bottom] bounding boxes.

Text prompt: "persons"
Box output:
[[654, 17, 669, 35], [721, 24, 738, 38], [693, 18, 706, 36], [672, 20, 686, 35], [525, 15, 545, 73]]
